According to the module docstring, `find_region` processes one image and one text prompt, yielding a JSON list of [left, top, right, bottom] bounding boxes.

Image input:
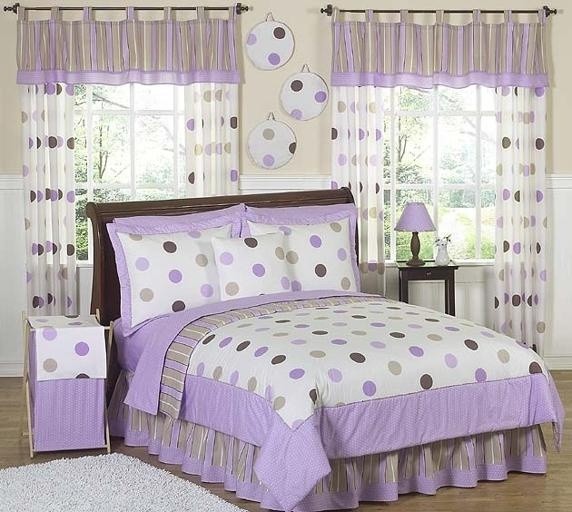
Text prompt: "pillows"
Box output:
[[106, 203, 360, 338]]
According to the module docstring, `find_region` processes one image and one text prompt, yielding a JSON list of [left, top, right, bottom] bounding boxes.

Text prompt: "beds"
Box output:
[[85, 186, 565, 512]]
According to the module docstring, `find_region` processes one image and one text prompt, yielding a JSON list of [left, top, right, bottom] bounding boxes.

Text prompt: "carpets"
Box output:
[[0, 452, 250, 512]]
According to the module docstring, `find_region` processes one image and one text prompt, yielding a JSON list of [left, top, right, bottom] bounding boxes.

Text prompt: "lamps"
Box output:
[[394, 202, 436, 265]]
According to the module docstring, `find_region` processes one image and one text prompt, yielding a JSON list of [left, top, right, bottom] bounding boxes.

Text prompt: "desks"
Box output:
[[396, 260, 458, 316]]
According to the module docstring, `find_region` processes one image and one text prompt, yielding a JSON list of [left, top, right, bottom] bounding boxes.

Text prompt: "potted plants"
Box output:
[[433, 231, 452, 266]]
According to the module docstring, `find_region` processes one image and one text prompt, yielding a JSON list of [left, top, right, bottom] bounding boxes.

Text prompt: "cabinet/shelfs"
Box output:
[[20, 309, 114, 458]]
